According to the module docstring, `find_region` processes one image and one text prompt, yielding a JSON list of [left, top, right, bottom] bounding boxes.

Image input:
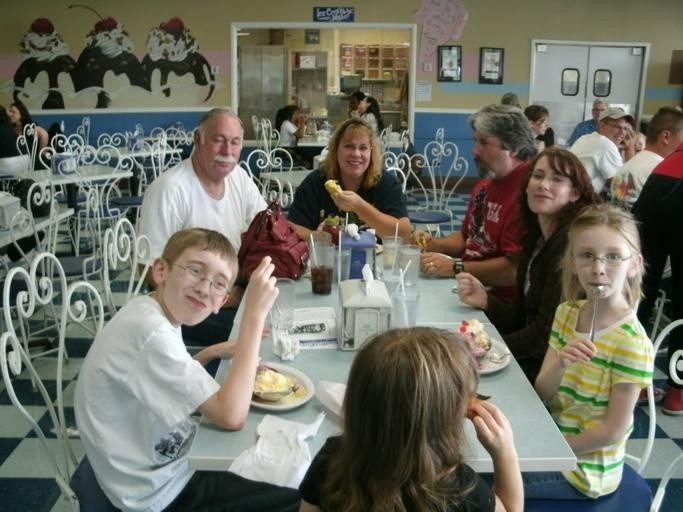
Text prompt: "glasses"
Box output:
[[604, 121, 630, 132], [169, 261, 231, 296], [571, 251, 632, 268]]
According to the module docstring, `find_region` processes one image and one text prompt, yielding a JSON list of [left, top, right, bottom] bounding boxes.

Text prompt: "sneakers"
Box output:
[[638, 385, 664, 403], [662, 385, 682, 416]]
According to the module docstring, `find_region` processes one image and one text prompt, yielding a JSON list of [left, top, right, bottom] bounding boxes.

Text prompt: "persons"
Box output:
[[132, 107, 287, 353], [73, 226, 301, 511], [497, 92, 683, 417], [0, 102, 21, 158], [299, 325, 526, 512], [287, 117, 409, 243], [480, 204, 655, 504], [276, 106, 310, 169], [348, 95, 386, 142], [455, 147, 602, 385], [410, 103, 537, 308], [8, 101, 49, 170], [346, 91, 365, 117]]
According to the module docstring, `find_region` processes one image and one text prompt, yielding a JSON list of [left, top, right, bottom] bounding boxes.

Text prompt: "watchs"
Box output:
[[453, 259, 465, 278]]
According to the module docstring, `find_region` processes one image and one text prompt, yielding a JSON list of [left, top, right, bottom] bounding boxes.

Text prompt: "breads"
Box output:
[[324, 177, 345, 193]]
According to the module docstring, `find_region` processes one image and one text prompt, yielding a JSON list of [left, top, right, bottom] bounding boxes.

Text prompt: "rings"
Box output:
[[429, 262, 434, 267]]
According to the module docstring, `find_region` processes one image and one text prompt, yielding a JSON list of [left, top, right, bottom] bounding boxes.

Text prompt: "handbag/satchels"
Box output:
[[237, 198, 310, 282]]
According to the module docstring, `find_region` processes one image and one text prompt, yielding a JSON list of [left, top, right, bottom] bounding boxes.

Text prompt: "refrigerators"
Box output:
[[238, 44, 287, 139]]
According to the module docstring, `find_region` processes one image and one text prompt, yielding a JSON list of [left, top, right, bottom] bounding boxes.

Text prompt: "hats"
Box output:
[[598, 106, 633, 122]]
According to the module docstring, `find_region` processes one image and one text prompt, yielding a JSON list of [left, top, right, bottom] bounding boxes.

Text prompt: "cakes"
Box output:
[[458, 316, 492, 361], [253, 367, 296, 401]]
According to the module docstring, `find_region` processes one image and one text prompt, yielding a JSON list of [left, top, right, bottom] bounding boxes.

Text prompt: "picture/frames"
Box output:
[[436, 45, 504, 84]]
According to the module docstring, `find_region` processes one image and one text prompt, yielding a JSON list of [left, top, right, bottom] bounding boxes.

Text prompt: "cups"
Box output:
[[308, 230, 419, 329], [271, 278, 295, 329]]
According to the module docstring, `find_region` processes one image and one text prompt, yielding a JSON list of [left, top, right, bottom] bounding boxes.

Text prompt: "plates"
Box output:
[[477, 337, 511, 372], [249, 361, 316, 412]]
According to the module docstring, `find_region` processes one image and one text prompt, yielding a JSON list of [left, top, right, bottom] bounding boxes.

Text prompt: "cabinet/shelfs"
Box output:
[[338, 43, 409, 83]]
